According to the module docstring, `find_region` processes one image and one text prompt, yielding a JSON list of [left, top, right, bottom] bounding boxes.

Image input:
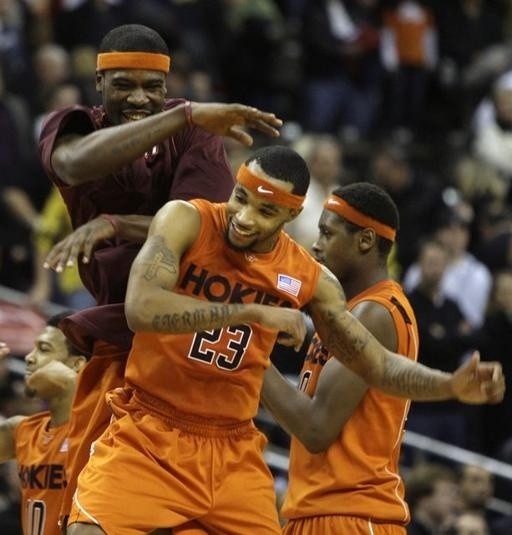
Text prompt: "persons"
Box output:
[[1, 24, 505, 535], [2, 1, 512, 503]]
